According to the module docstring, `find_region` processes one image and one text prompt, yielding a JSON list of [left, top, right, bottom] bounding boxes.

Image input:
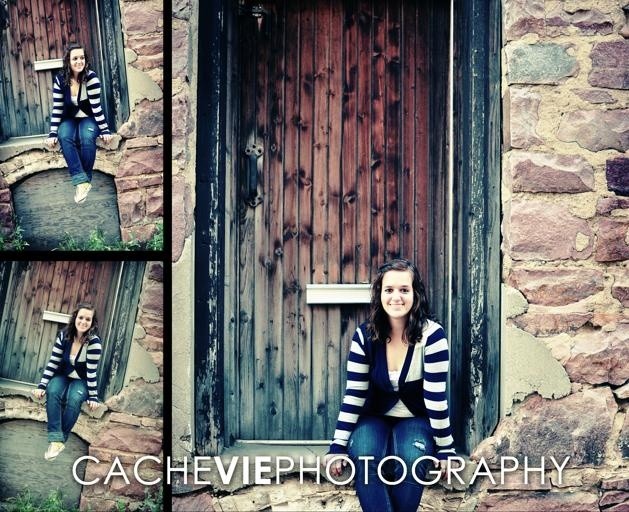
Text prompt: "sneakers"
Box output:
[[74, 183, 92, 204], [44, 442, 65, 462]]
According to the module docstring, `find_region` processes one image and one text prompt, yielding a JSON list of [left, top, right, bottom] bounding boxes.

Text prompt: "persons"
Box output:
[[46, 43, 112, 205], [322, 260, 459, 512], [31, 304, 102, 462]]
